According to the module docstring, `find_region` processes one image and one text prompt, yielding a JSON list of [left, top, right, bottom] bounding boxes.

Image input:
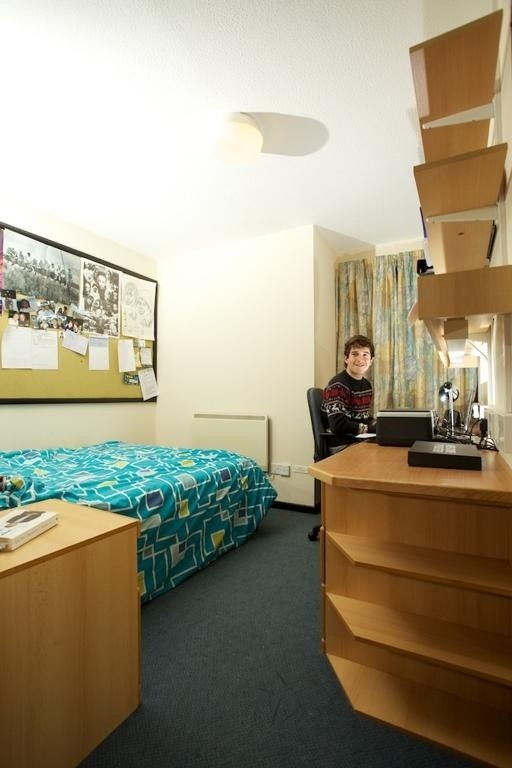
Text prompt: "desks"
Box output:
[[0, 497, 142, 768], [307, 433, 510, 767]]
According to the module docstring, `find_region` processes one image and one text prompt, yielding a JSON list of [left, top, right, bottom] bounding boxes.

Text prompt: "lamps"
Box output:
[[439, 380, 459, 443], [440, 315, 469, 359]]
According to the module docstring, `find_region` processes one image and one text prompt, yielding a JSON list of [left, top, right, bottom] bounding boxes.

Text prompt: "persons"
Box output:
[[319, 334, 377, 455], [0, 251, 119, 339], [4, 510, 46, 528]]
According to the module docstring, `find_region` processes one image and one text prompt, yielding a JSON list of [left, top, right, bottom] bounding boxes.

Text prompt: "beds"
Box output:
[[0, 437, 262, 606]]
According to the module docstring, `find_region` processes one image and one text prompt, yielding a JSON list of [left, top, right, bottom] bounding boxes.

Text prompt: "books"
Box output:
[[0, 508, 58, 551]]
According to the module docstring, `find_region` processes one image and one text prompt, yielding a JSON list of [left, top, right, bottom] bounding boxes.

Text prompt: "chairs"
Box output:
[[305, 386, 362, 540]]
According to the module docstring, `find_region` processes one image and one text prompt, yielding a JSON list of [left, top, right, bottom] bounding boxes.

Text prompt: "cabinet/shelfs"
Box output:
[[407, 3, 510, 369], [321, 527, 510, 766]]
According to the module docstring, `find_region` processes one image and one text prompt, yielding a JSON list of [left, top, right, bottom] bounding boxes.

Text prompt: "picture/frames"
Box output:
[[0, 220, 157, 405]]
